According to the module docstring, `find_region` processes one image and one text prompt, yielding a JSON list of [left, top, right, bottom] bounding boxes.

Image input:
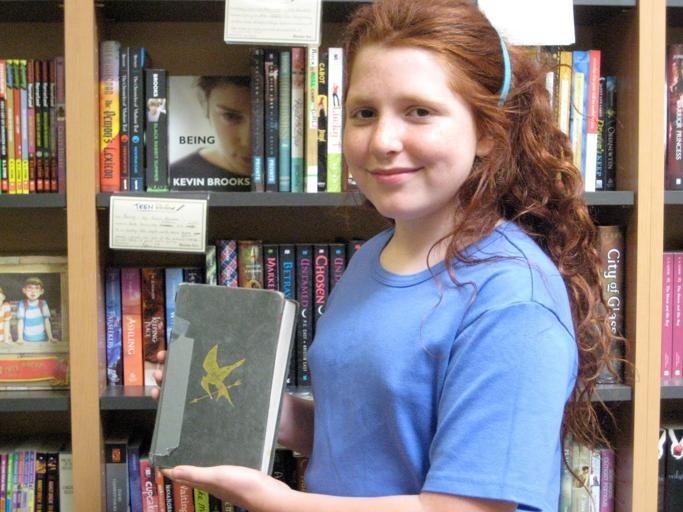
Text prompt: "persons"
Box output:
[[158, 1, 612, 512], [331, 81, 340, 106], [16, 277, 57, 343], [0, 289, 15, 346]]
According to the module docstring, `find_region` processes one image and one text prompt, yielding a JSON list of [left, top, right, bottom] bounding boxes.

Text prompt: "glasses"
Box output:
[[25, 285, 42, 292]]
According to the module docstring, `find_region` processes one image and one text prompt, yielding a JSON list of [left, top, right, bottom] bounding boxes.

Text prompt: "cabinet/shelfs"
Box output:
[[1, 2, 683, 511]]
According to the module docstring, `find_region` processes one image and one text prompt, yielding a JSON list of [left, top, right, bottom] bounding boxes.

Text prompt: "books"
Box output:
[[515, 48, 616, 189], [101, 424, 247, 512], [329, 241, 346, 294], [0, 255, 69, 389], [217, 239, 238, 287], [560, 429, 613, 512], [121, 46, 130, 194], [99, 40, 120, 192], [270, 448, 310, 491], [278, 244, 296, 387], [122, 267, 144, 387], [249, 49, 265, 192], [313, 244, 330, 340], [305, 46, 317, 193], [660, 420, 683, 512], [290, 47, 304, 192], [661, 253, 683, 378], [595, 225, 626, 385], [339, 236, 366, 267], [129, 45, 153, 193], [183, 268, 206, 283], [286, 226, 313, 386], [0, 57, 67, 193], [264, 52, 279, 192], [165, 268, 184, 347], [106, 265, 122, 385], [152, 282, 299, 475], [239, 240, 263, 289], [144, 69, 169, 193], [141, 267, 167, 387], [328, 47, 344, 192], [204, 245, 217, 285], [264, 243, 280, 291], [279, 51, 291, 191], [317, 51, 327, 192], [0, 432, 76, 512], [663, 44, 683, 194]]
